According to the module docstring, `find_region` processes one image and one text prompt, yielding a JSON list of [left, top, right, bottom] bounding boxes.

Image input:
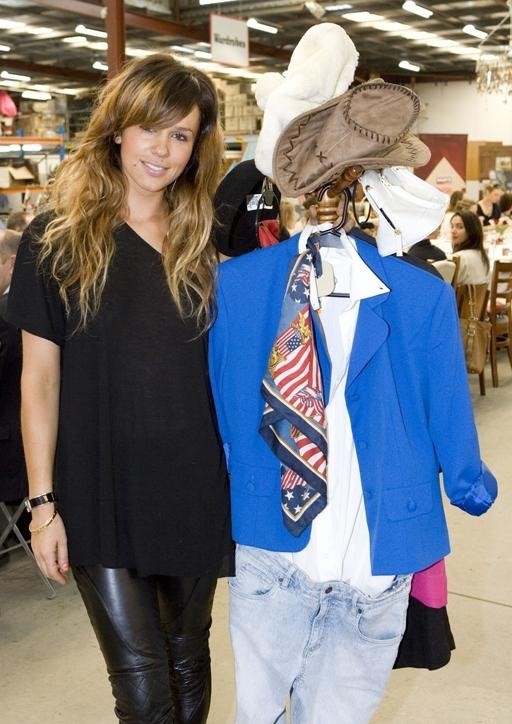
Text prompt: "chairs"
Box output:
[[456, 259, 511, 396]]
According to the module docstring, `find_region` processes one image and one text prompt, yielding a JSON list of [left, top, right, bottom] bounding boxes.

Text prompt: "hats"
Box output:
[[272, 79, 431, 198], [211, 159, 281, 258]]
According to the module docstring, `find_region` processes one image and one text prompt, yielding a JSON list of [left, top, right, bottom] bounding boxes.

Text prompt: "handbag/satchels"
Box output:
[[460, 318, 491, 374]]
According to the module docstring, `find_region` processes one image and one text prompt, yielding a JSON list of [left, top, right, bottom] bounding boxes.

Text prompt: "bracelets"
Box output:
[[28, 511, 58, 533], [25, 492, 57, 513]]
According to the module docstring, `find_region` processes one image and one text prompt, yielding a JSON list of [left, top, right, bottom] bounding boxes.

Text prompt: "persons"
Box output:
[[7, 211, 37, 234], [407, 180, 511, 286], [0, 51, 357, 723], [0, 228, 38, 570]]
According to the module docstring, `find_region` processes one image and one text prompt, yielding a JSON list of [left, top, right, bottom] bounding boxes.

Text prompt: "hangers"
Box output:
[[307, 184, 355, 297]]
